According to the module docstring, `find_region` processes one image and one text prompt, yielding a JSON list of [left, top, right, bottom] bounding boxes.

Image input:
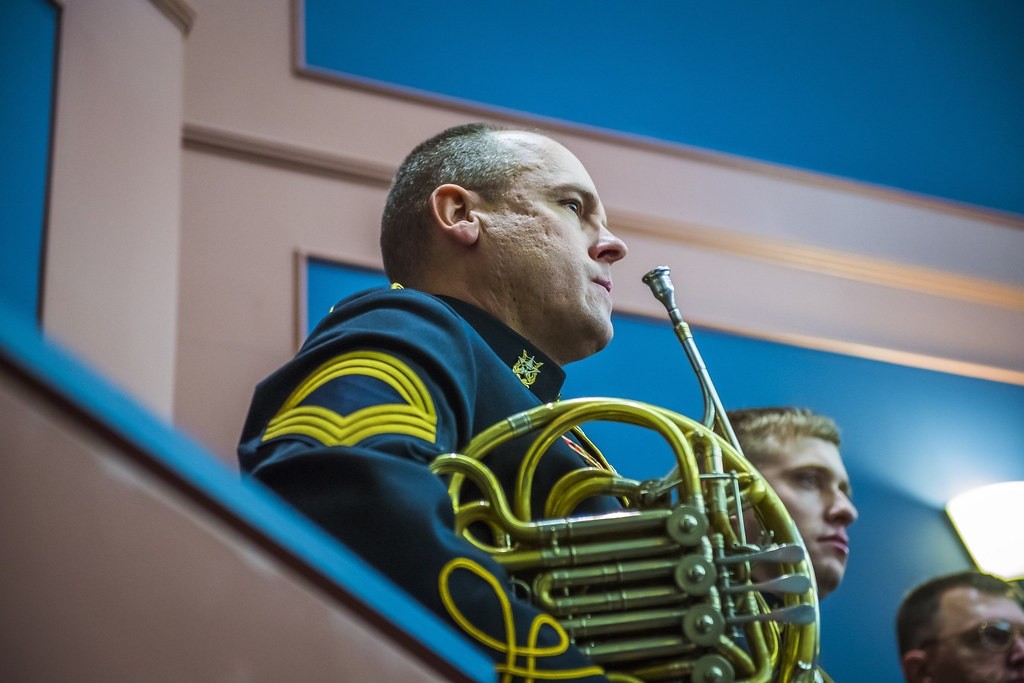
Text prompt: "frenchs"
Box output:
[[425, 264, 826, 682]]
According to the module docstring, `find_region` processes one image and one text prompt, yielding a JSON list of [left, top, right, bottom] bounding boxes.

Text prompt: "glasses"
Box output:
[[918, 622, 1024, 654]]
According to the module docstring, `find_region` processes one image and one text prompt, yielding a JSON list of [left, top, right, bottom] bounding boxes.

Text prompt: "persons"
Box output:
[[235, 119, 724, 683], [895, 569, 1024, 683], [677, 405, 861, 683]]
